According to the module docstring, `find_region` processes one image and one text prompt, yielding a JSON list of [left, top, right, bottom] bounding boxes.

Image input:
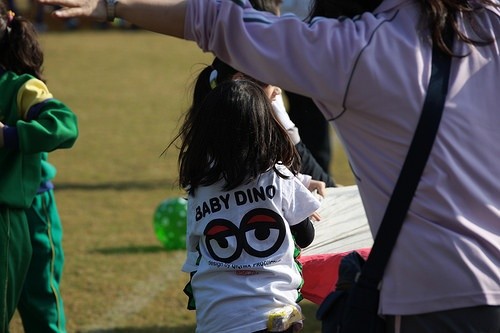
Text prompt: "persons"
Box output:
[[0, 0, 78, 333], [184, 82, 323, 333], [178, 57, 326, 310], [38, 0, 500, 333], [249, 0, 343, 188]]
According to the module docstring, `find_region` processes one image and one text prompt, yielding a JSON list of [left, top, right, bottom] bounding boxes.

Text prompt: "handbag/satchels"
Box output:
[[316, 250, 380, 332]]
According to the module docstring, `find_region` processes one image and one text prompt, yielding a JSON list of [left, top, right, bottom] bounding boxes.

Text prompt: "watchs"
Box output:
[[104, 0, 119, 22]]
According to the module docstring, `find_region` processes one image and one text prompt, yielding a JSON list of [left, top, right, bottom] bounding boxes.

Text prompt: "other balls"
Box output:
[[152, 196, 188, 249]]
